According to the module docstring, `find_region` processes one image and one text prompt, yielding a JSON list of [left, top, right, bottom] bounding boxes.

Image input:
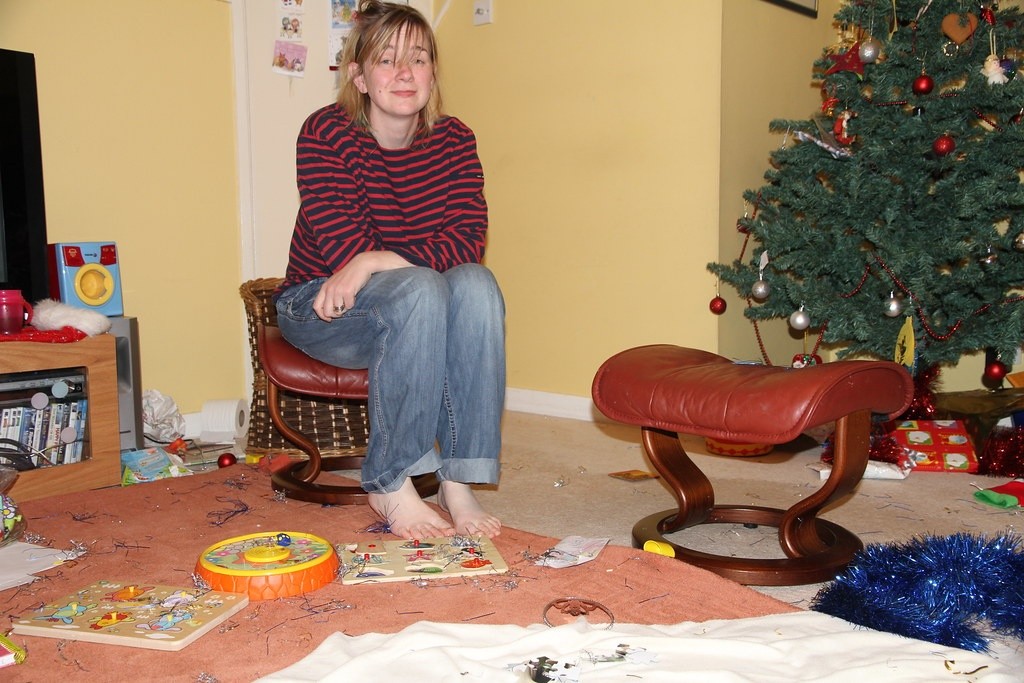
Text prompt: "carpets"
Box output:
[[0, 460, 804, 683]]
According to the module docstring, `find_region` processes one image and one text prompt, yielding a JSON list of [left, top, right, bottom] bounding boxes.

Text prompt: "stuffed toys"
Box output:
[[834, 112, 856, 143], [979, 54, 1010, 84]]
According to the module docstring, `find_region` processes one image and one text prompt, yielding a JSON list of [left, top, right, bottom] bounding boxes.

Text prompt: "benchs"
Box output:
[[589, 345, 915, 588]]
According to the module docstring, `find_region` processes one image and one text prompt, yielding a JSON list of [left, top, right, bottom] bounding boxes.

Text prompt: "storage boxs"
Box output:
[[47, 242, 125, 318], [883, 419, 979, 476], [0, 334, 122, 506]]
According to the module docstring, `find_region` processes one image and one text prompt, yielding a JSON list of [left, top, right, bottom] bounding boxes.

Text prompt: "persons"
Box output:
[[274, 0, 507, 539], [77, 411, 82, 419]]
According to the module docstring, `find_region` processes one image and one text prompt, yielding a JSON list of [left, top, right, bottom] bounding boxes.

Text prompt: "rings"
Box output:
[[333, 304, 345, 312]]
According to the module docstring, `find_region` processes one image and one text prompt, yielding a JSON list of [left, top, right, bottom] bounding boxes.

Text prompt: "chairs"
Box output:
[[238, 277, 439, 504]]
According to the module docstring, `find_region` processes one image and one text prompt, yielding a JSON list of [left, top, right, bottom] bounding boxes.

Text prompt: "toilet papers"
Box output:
[[199, 397, 250, 443]]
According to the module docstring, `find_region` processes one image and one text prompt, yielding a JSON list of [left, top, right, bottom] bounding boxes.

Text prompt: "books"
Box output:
[[335, 536, 509, 584], [0, 374, 89, 466], [0, 632, 25, 670], [11, 578, 248, 651]]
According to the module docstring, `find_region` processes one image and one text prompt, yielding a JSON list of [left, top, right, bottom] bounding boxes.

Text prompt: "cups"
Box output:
[[0, 289, 33, 335]]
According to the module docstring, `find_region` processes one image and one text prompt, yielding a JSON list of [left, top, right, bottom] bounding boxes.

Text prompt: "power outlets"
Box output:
[[473, 1, 493, 27]]
